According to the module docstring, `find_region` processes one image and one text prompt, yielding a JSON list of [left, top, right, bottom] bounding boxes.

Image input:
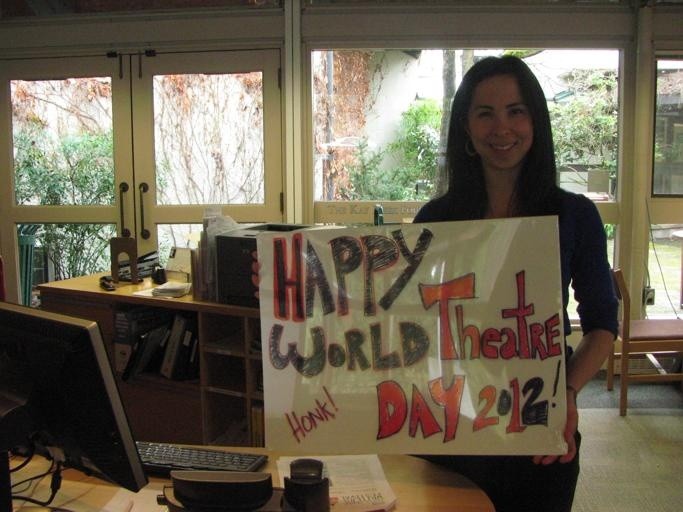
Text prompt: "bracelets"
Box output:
[[566, 384, 578, 397]]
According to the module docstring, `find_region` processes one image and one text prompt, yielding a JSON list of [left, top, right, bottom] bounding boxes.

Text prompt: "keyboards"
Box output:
[[134, 441, 269, 472]]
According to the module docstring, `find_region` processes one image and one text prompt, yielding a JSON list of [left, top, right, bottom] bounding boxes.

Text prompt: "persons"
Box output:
[[411, 55, 619, 511]]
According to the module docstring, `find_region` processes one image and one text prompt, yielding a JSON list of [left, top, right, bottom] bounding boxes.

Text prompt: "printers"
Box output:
[[214, 222, 348, 310]]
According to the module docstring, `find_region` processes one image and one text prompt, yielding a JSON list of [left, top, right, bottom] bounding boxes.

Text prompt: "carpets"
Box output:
[[575, 367, 683, 409]]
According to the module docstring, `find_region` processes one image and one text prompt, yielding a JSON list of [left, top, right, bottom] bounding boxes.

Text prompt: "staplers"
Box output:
[[99, 276, 114, 291]]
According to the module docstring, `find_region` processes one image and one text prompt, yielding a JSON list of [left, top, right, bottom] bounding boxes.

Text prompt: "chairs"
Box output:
[[607, 267, 683, 416]]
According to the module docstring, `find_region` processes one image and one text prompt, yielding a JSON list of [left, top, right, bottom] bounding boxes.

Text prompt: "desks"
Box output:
[[7, 441, 495, 511]]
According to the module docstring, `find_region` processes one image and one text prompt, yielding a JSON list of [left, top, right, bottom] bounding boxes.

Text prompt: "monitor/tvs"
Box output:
[[0, 302, 149, 512]]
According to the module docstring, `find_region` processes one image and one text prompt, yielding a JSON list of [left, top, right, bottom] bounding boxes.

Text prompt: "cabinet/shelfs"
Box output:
[[37, 272, 265, 447]]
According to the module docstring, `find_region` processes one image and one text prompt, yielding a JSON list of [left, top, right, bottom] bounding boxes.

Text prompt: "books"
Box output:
[[119, 246, 192, 296]]
